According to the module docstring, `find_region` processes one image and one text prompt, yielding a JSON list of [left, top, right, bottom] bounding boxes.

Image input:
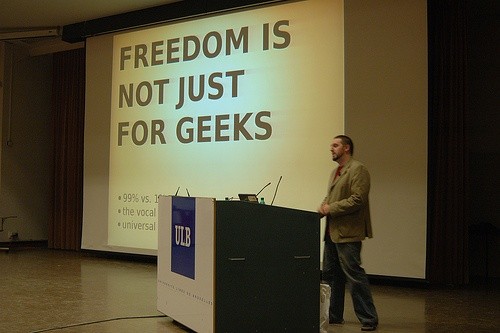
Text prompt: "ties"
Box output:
[[335, 166, 342, 178]]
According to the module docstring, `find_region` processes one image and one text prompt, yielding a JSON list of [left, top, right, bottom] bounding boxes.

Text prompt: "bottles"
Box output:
[[260, 197, 265, 204]]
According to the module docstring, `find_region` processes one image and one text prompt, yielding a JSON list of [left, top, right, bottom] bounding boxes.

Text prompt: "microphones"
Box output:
[[271, 176, 282, 205], [256, 183, 271, 196]]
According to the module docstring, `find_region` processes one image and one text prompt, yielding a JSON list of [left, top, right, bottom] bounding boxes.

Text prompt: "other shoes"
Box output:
[[361, 325, 376, 332], [328, 320, 345, 326]]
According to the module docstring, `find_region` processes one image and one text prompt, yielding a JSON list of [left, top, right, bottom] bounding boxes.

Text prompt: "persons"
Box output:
[[317, 135, 381, 331]]
